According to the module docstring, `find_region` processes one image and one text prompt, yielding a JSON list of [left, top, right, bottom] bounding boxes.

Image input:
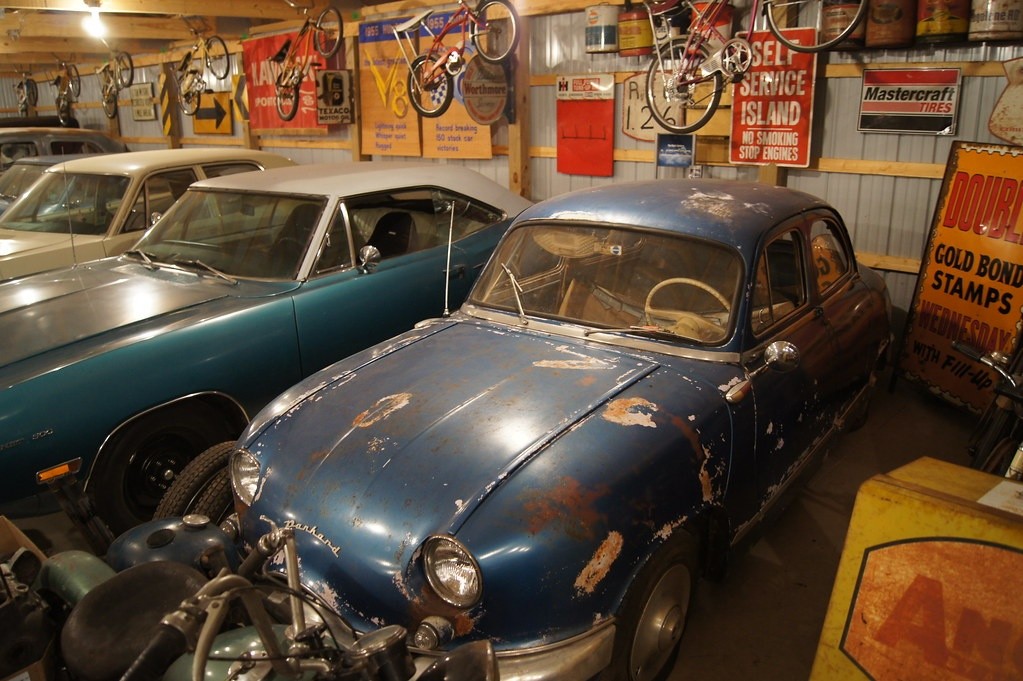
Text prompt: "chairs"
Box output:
[[267, 202, 344, 274], [567, 261, 685, 329], [354, 211, 410, 265], [688, 247, 772, 332]]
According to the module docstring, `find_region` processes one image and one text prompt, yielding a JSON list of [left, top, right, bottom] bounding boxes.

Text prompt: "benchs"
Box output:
[[337, 208, 485, 262]]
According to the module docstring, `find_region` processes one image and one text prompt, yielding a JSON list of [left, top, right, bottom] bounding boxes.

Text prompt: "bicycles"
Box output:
[[16, 71, 39, 120], [389, 0, 522, 119], [172, 13, 230, 117], [49, 60, 81, 127], [93, 49, 134, 120], [641, 0, 869, 136], [266, 0, 343, 121]]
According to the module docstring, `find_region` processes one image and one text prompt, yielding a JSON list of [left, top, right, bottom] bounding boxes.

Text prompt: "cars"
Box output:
[[0, 115, 640, 573], [147, 174, 905, 681]]
